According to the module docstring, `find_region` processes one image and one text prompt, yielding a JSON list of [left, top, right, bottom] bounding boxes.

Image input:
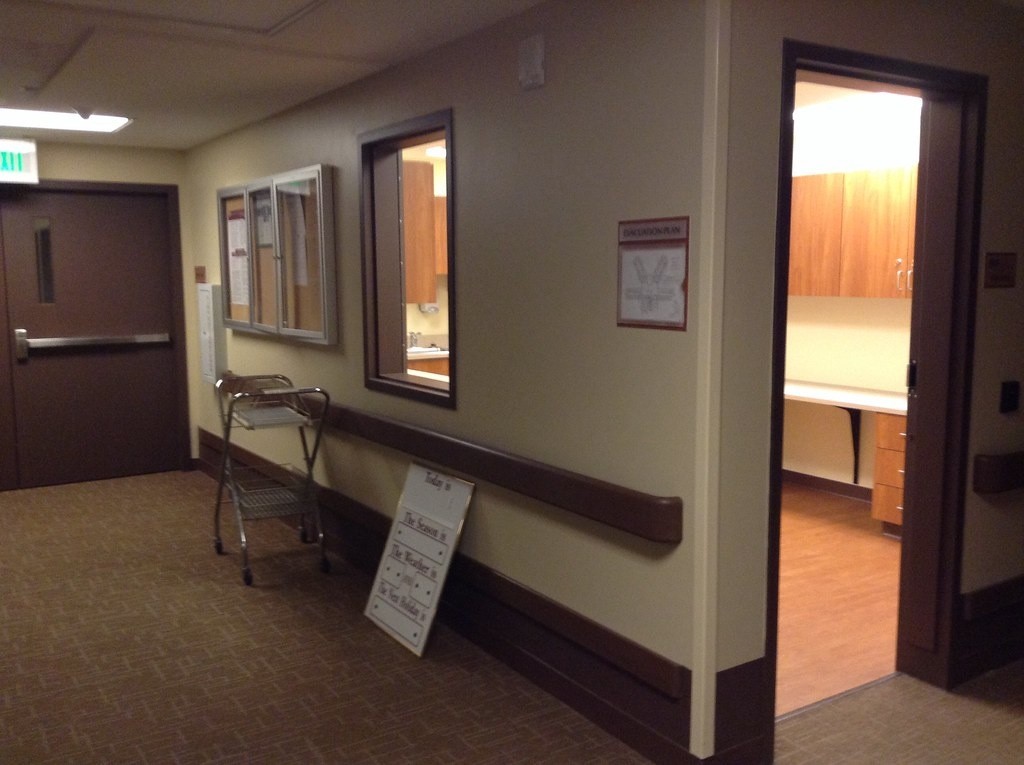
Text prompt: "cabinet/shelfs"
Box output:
[[783, 167, 920, 300], [870, 412, 907, 538]]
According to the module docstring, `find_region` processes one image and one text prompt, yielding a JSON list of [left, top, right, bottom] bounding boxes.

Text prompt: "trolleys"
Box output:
[[212, 372, 330, 586]]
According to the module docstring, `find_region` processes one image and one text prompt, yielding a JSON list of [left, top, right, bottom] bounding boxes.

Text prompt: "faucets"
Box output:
[[408, 332, 421, 347]]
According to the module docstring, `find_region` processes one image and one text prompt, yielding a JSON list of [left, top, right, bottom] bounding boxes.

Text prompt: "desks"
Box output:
[[781, 379, 909, 484]]
